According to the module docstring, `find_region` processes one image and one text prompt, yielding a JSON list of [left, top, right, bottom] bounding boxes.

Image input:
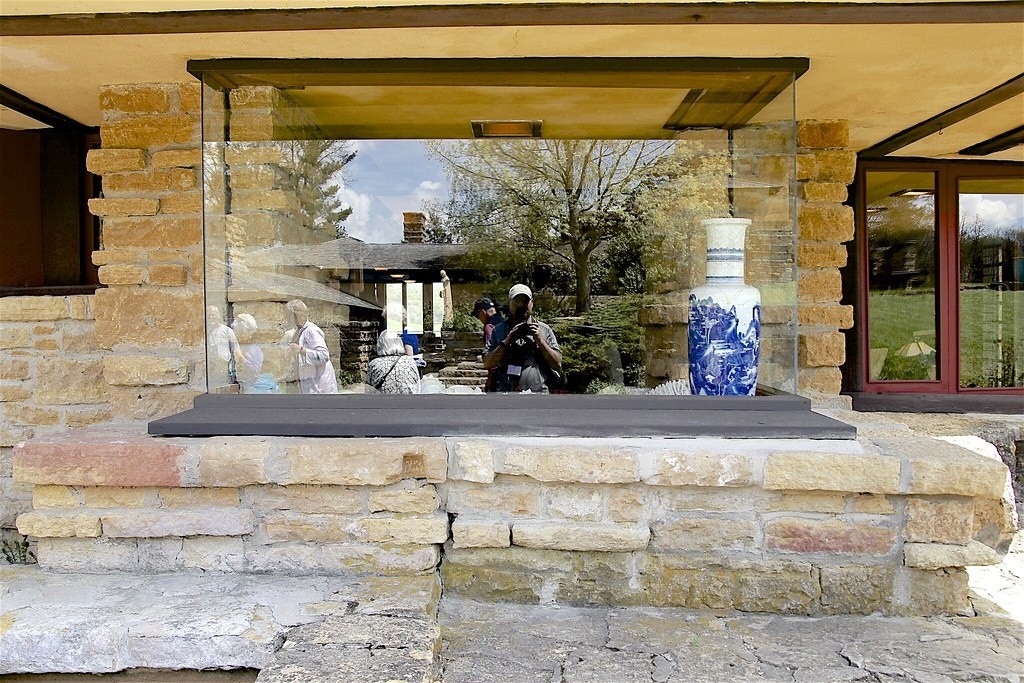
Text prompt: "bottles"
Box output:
[[688, 218, 761, 396]]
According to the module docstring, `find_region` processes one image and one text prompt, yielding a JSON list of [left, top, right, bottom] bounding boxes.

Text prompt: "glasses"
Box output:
[[475, 309, 487, 319]]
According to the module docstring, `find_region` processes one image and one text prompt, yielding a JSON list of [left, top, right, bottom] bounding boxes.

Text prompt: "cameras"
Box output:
[[517, 324, 534, 335]]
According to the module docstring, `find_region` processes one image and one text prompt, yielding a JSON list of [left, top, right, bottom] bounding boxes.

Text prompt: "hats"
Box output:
[[470, 298, 494, 316], [509, 283, 532, 299]]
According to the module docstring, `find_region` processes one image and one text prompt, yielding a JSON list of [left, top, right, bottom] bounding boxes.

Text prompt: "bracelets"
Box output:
[[499, 340, 508, 351]]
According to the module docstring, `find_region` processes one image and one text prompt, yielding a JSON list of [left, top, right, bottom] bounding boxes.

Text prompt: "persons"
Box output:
[[282, 299, 338, 394], [364, 302, 424, 394], [206, 305, 265, 395], [481, 284, 562, 392], [470, 297, 510, 392]]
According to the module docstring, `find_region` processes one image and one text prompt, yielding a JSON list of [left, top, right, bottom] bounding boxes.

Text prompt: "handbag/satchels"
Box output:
[[211, 359, 231, 384], [546, 368, 561, 385]]
